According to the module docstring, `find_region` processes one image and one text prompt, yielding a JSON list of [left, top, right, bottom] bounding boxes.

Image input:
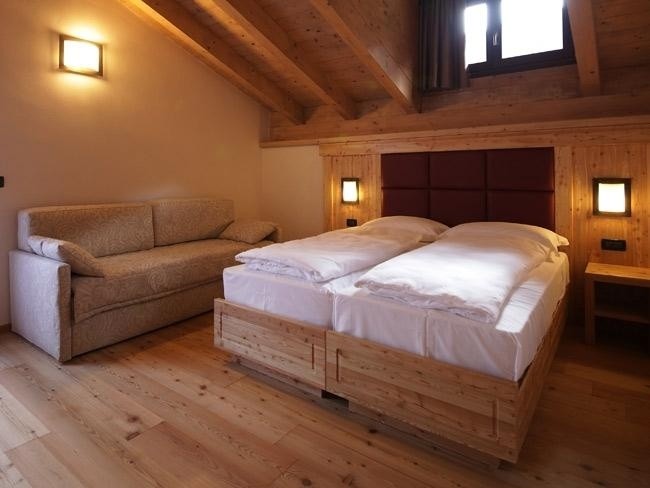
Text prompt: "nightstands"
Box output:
[[582, 262, 648, 351]]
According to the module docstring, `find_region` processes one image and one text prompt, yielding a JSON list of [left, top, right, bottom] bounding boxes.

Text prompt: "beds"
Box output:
[[212, 225, 570, 470]]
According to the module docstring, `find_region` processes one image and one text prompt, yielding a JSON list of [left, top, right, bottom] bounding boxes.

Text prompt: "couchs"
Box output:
[[9, 197, 281, 360]]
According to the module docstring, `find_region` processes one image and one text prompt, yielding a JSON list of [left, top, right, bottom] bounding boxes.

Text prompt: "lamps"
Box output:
[[56, 34, 104, 78], [340, 177, 360, 205], [589, 178, 632, 216]]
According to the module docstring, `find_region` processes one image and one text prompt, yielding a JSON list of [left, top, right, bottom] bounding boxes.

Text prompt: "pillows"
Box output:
[[361, 214, 569, 252]]
